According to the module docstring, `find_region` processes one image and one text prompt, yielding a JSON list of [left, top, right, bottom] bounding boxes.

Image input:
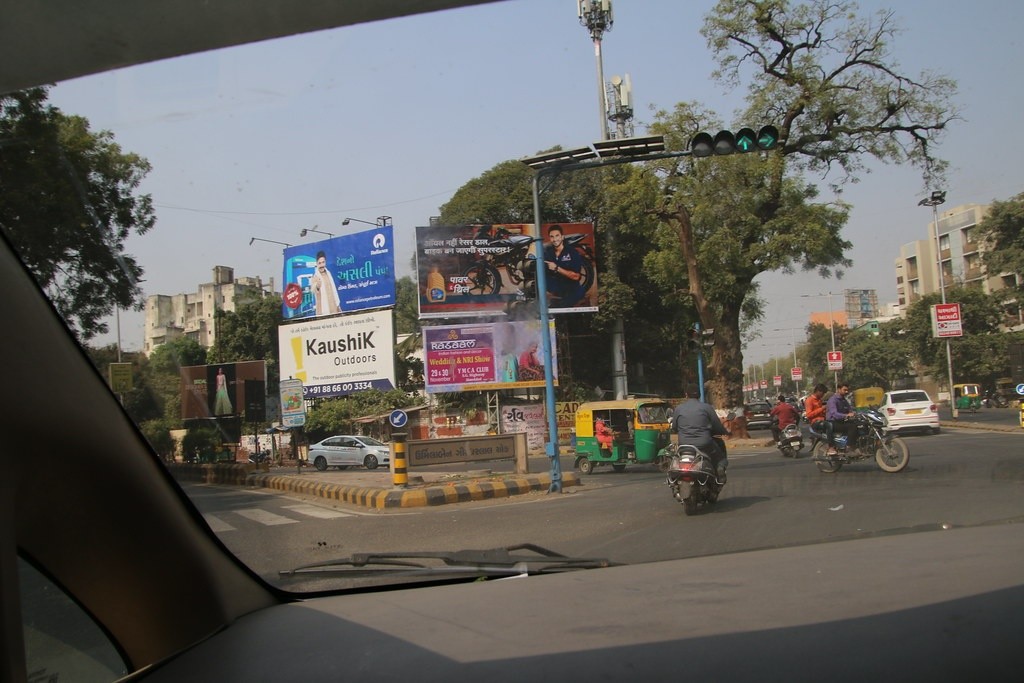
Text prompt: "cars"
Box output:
[[307, 434, 391, 472], [873, 389, 941, 437], [744, 397, 808, 431]]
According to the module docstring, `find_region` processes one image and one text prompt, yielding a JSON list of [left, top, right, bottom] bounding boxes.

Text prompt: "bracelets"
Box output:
[[555, 266, 559, 271]]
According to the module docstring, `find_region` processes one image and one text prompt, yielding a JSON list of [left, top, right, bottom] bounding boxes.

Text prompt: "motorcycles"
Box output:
[[765, 411, 806, 459], [247, 444, 274, 468], [807, 404, 910, 473], [666, 412, 738, 516], [459, 225, 595, 303], [571, 396, 673, 476], [953, 383, 985, 417]]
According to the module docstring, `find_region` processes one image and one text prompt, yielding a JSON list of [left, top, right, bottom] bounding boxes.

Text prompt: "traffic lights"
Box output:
[[686, 328, 703, 354], [689, 123, 780, 158], [700, 327, 717, 350]]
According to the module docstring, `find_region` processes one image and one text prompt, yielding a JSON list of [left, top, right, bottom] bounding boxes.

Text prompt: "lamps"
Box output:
[[300, 229, 333, 239], [249, 237, 292, 248], [342, 218, 381, 228]]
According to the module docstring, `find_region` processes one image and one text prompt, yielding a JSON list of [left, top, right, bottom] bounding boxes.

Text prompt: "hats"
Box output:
[[686, 383, 701, 398]]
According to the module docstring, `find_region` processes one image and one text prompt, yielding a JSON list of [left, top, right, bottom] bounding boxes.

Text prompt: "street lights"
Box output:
[[799, 291, 860, 393], [771, 327, 805, 396], [917, 189, 958, 419], [760, 343, 792, 397]]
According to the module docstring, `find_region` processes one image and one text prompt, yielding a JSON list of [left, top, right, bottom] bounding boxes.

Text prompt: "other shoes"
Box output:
[[718, 466, 727, 483], [827, 449, 837, 456], [845, 448, 857, 455]]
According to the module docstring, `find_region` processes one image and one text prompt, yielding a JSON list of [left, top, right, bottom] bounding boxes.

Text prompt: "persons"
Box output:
[[310, 252, 341, 316], [672, 383, 728, 484], [827, 382, 860, 456], [628, 413, 641, 438], [595, 413, 619, 454], [519, 226, 585, 306], [766, 396, 804, 448], [214, 367, 233, 414], [519, 342, 545, 380], [805, 384, 838, 455]]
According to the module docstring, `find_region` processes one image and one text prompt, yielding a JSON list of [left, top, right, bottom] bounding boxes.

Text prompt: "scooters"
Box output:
[[985, 384, 1008, 408]]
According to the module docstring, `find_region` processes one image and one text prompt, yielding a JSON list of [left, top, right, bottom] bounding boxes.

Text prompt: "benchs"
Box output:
[[619, 432, 634, 448]]
